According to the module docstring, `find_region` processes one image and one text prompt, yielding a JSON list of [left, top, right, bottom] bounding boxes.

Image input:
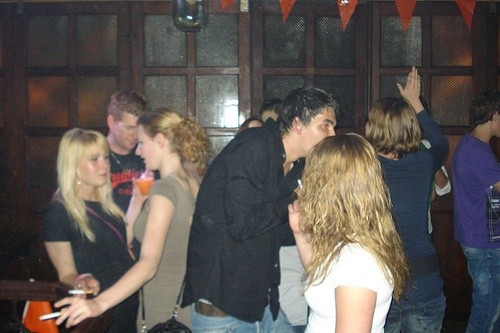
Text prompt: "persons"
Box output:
[[180, 85, 339, 333], [40, 66, 500, 333]]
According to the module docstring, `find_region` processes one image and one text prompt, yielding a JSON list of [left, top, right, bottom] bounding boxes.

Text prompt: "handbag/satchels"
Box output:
[[145, 316, 192, 333]]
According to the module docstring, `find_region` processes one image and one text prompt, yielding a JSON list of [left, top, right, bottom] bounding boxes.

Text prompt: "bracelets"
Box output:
[[73, 272, 93, 284]]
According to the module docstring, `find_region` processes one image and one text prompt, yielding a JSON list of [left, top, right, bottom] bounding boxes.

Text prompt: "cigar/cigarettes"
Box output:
[[68, 289, 93, 294], [297, 178, 302, 189], [41, 312, 63, 320]]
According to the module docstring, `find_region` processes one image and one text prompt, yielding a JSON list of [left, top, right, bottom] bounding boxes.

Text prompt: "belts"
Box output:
[[194, 301, 230, 317]]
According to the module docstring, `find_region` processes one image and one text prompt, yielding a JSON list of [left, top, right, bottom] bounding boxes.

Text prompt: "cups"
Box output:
[[132, 170, 155, 196]]
